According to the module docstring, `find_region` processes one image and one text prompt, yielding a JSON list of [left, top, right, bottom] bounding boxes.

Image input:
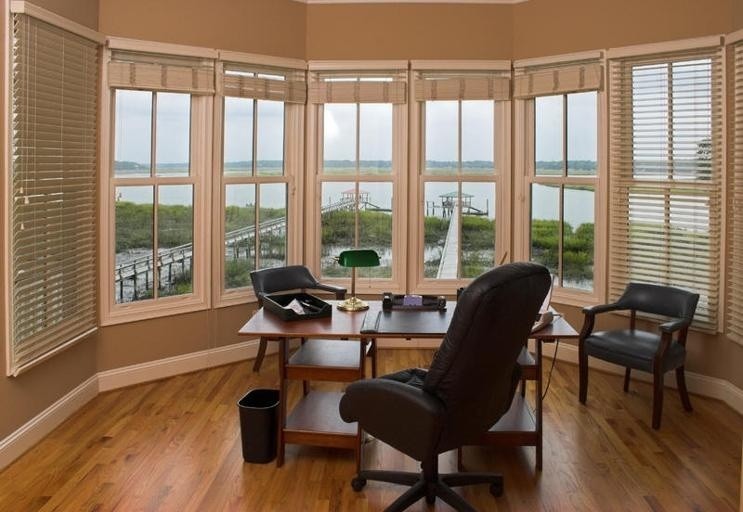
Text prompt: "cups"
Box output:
[[438, 296, 446, 309]]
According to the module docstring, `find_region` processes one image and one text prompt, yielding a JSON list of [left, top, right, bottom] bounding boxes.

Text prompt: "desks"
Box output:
[[238, 300, 580, 474]]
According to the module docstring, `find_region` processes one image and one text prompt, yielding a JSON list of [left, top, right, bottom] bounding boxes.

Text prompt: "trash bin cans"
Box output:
[[236, 389, 279, 464]]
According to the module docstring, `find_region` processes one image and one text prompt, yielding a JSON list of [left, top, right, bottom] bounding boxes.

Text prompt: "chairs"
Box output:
[[339, 263, 551, 512], [251, 266, 347, 391], [578, 282, 699, 430]]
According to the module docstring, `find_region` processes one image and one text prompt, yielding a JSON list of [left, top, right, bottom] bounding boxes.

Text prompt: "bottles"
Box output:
[[382, 292, 393, 313]]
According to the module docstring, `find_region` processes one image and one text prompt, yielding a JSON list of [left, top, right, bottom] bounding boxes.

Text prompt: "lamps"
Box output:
[[332, 250, 381, 312]]
